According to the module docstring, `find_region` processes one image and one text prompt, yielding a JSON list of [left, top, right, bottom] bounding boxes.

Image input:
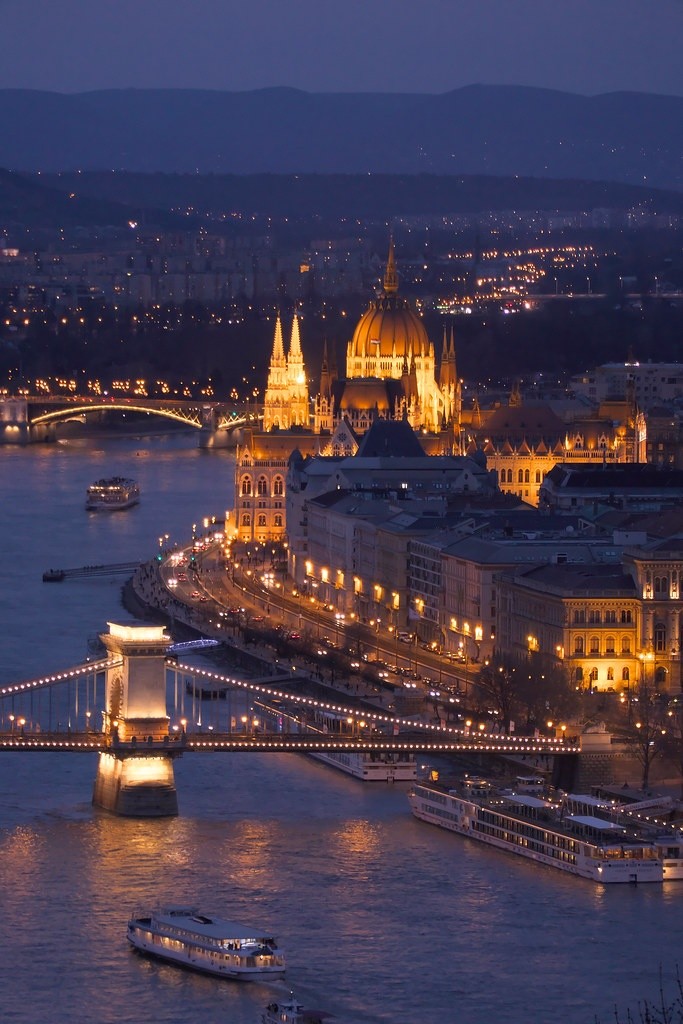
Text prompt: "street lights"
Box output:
[[235, 562, 244, 597], [433, 642, 442, 682], [328, 605, 339, 643], [409, 633, 417, 672], [293, 591, 301, 628], [311, 598, 319, 638], [371, 617, 381, 661], [277, 583, 285, 621], [351, 613, 359, 664], [247, 570, 255, 607]]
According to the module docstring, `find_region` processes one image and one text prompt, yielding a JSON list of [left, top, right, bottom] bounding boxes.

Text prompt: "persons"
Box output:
[[49, 564, 103, 573], [198, 624, 442, 726], [235, 556, 279, 567], [520, 755, 551, 768], [133, 557, 194, 624]]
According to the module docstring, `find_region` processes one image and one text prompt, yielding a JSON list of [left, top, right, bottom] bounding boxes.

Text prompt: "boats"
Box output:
[[251, 695, 418, 782], [261, 998, 334, 1024], [84, 476, 142, 510], [127, 901, 286, 984], [514, 775, 544, 793], [459, 774, 490, 795], [406, 773, 666, 885], [177, 542, 212, 583]]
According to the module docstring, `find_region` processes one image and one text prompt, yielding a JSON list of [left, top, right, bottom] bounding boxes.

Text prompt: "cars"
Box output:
[[649, 693, 668, 704], [229, 608, 239, 614], [319, 635, 499, 716], [287, 631, 301, 641], [667, 695, 683, 709], [200, 596, 207, 602], [252, 615, 264, 623], [275, 624, 284, 632], [192, 590, 200, 598]]
